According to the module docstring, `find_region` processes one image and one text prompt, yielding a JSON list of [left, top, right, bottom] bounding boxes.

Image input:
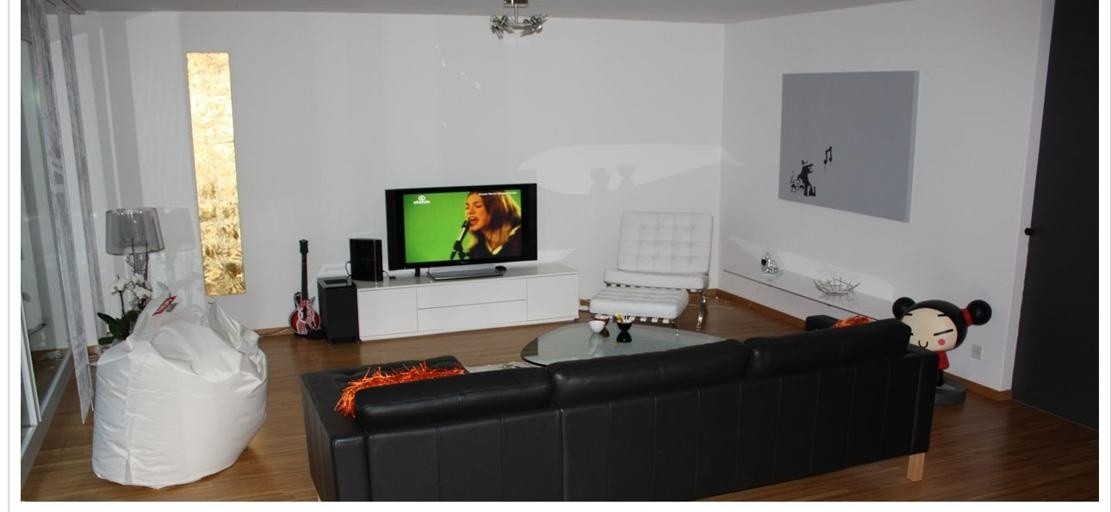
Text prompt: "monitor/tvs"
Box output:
[[385, 183, 538, 275]]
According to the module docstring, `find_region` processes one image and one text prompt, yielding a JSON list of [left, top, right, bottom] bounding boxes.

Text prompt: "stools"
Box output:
[[589, 284, 691, 327]]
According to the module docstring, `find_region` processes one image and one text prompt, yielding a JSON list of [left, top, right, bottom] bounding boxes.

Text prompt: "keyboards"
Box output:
[[428, 269, 504, 281]]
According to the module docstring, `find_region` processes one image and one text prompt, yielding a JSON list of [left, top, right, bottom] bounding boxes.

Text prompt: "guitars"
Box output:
[[289, 239, 321, 336]]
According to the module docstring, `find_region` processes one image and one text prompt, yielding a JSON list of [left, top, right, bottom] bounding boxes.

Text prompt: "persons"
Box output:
[[888, 295, 991, 390], [463, 188, 524, 260]]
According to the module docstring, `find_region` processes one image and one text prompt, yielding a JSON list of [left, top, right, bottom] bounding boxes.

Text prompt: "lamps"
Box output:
[[103, 205, 166, 286]]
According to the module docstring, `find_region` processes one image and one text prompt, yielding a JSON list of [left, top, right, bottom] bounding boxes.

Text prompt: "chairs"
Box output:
[[588, 212, 714, 333]]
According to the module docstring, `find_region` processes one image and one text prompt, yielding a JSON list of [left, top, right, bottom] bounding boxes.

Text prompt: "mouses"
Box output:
[[495, 266, 507, 271]]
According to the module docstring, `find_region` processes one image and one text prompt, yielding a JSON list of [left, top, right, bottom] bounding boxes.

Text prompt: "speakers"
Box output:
[[317, 277, 358, 345], [349, 238, 383, 281]]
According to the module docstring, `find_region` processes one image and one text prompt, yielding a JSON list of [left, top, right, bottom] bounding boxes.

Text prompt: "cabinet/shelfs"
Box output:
[[353, 266, 582, 341]]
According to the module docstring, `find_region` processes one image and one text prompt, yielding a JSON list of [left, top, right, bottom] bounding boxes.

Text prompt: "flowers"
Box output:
[[93, 275, 147, 345]]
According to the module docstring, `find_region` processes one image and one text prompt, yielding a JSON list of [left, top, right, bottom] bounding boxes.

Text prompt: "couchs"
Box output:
[[299, 318, 940, 501]]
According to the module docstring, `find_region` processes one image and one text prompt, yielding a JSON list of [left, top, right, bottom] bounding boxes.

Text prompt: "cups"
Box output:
[[589, 313, 632, 333], [599, 327, 632, 343]]
